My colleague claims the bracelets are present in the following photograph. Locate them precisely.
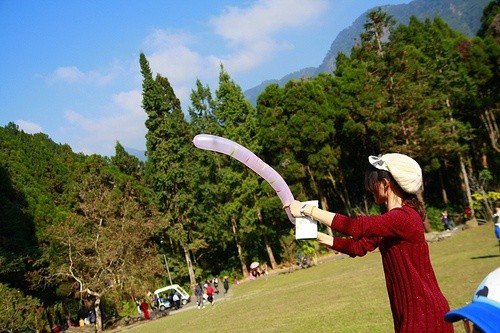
[310,204,316,221]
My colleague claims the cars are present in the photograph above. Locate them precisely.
[153,284,190,311]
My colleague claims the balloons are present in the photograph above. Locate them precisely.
[192,134,297,225]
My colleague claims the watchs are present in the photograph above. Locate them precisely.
[300,203,309,217]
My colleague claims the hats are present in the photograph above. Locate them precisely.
[445,267,500,333]
[368,153,424,193]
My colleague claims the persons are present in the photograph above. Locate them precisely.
[233,275,239,284]
[195,282,205,309]
[250,262,268,280]
[444,266,500,333]
[207,283,213,304]
[224,278,228,294]
[297,251,308,265]
[492,206,500,240]
[460,202,472,225]
[141,299,151,320]
[441,209,458,230]
[37,307,98,333]
[197,276,219,303]
[161,290,181,311]
[137,303,143,314]
[282,153,454,333]
